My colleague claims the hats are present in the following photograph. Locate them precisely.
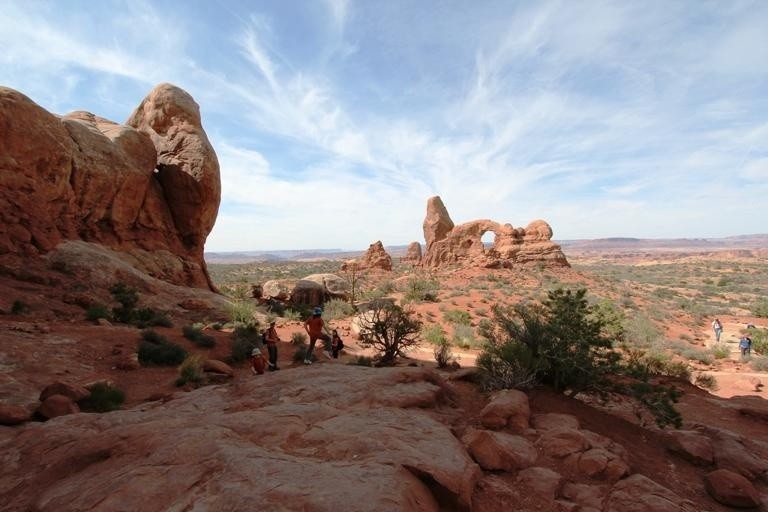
[251,348,262,356]
[313,307,322,315]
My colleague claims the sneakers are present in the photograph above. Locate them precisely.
[304,359,312,365]
[270,367,280,371]
[322,350,331,359]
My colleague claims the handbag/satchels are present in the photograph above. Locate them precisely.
[337,336,344,350]
[262,329,271,344]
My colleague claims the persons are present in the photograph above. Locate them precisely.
[739,334,752,356]
[264,323,281,370]
[711,318,724,342]
[303,308,331,365]
[330,330,340,360]
[248,349,274,376]
